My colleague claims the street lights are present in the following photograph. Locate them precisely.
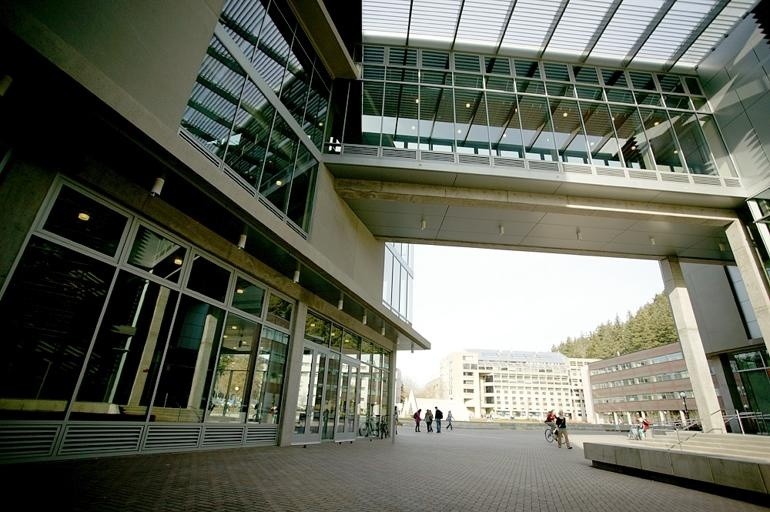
[680,392,690,425]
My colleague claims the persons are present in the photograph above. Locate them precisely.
[395,406,455,434]
[555,410,573,449]
[545,410,559,441]
[635,412,646,440]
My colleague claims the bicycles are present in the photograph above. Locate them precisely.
[545,424,566,444]
[359,414,389,437]
